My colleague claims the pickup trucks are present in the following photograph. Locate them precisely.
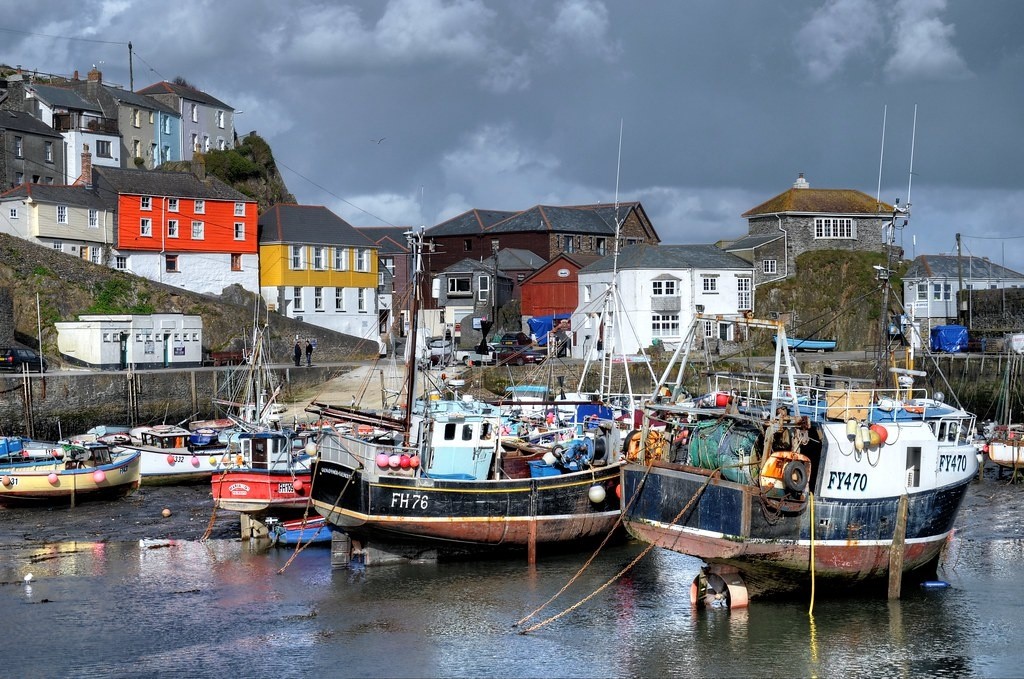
[453,342,503,367]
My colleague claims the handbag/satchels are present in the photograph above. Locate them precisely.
[292,355,296,361]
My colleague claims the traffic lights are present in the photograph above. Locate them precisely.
[446,330,451,341]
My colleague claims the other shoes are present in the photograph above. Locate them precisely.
[295,364,300,366]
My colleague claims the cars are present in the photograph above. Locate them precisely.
[500,332,533,348]
[0,347,49,375]
[489,345,547,367]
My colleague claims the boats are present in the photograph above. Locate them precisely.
[210,290,430,541]
[619,105,983,609]
[307,230,697,569]
[0,291,297,511]
[263,514,332,548]
[772,333,837,350]
[301,221,1024,482]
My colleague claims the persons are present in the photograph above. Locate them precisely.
[295,342,302,366]
[589,414,600,429]
[306,341,313,367]
